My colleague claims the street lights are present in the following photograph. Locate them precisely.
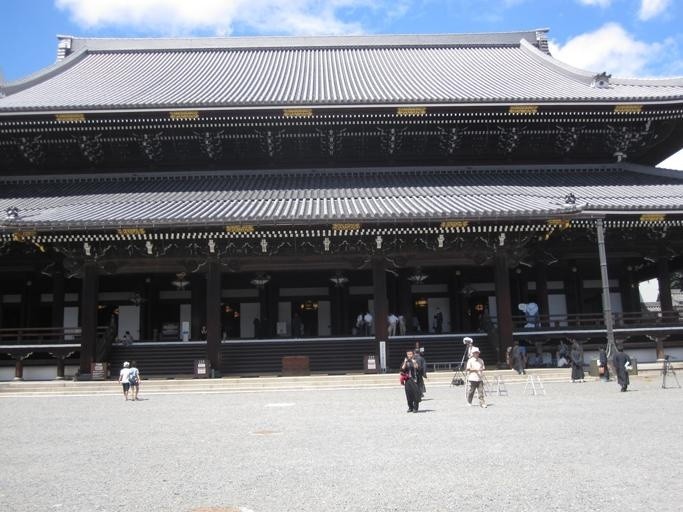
[598,367,605,374]
[128,376,138,385]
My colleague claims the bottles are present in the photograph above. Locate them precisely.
[123,361,130,367]
[472,347,481,354]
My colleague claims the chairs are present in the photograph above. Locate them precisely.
[466,401,473,407]
[412,408,418,413]
[480,404,487,408]
[406,408,413,412]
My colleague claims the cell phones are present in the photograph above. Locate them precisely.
[406,359,412,362]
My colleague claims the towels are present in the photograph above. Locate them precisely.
[363,350,379,374]
[193,358,211,379]
[90,360,108,381]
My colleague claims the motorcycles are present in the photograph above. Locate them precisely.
[451,344,468,387]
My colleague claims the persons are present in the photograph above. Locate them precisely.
[464,347,488,408]
[126,331,133,346]
[123,335,127,344]
[524,299,540,327]
[400,350,420,413]
[354,307,443,336]
[119,361,130,400]
[201,325,208,340]
[505,334,632,392]
[412,347,426,402]
[129,360,141,401]
